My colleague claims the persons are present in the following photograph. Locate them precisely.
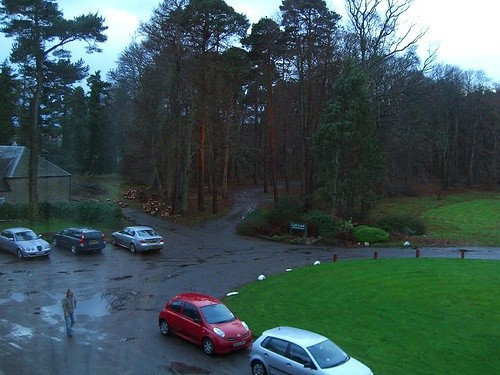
[62,288,77,337]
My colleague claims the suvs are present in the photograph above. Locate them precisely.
[52,227,106,256]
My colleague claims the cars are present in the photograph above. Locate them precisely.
[0,227,51,258]
[159,292,252,356]
[111,226,164,254]
[248,326,373,375]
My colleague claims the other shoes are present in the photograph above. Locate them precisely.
[67,333,72,338]
